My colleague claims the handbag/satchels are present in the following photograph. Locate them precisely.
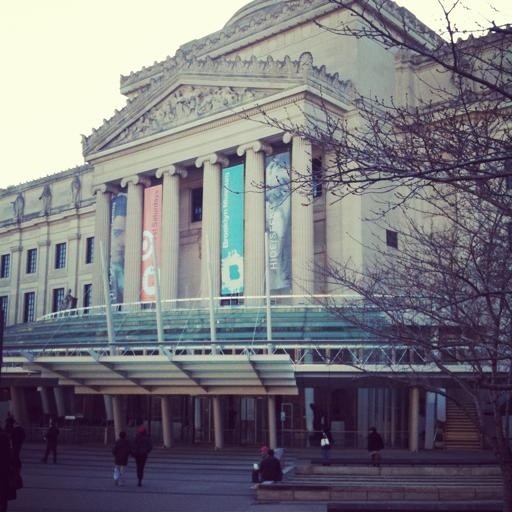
[116,440,139,457]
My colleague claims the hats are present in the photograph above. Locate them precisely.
[257,447,270,453]
[136,425,145,433]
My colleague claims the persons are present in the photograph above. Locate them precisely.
[0,430,23,511]
[132,424,152,486]
[367,427,385,466]
[112,431,129,487]
[4,411,13,439]
[11,423,25,450]
[320,424,332,466]
[309,401,325,430]
[41,418,60,464]
[260,449,283,482]
[248,445,268,491]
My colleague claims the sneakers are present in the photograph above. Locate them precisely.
[115,478,142,488]
[249,485,259,490]
